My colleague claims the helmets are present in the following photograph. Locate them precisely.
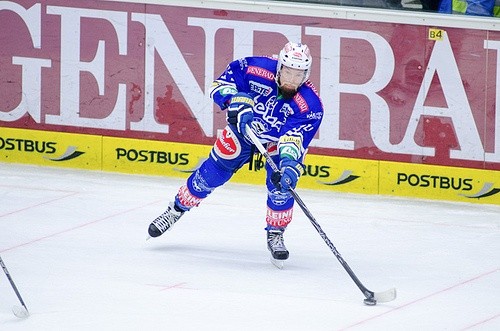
[278,42,312,85]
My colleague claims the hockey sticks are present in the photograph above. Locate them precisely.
[245,123,396,302]
[0,257,30,317]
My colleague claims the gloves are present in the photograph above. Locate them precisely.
[226,93,255,137]
[269,161,304,192]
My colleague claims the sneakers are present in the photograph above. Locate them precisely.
[263,226,290,269]
[146,204,185,237]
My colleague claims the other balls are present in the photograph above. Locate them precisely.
[364,299,376,305]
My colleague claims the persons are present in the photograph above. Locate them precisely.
[146,42,324,270]
